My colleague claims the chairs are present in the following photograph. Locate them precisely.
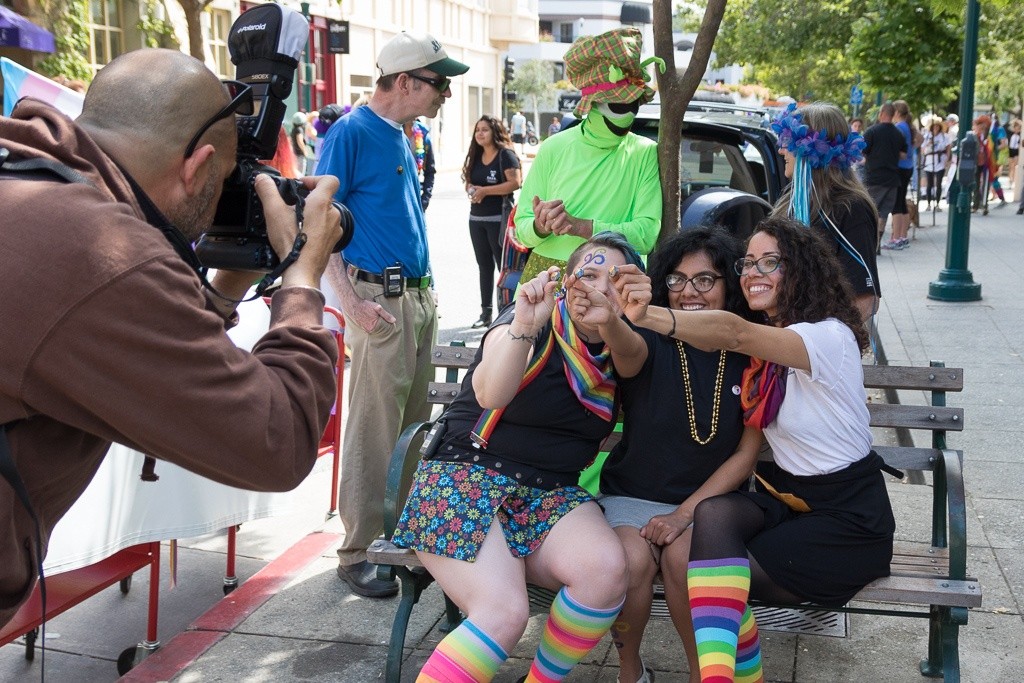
[731,160,770,204]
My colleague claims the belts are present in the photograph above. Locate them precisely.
[343,259,432,288]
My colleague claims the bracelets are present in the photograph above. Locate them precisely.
[666,307,677,338]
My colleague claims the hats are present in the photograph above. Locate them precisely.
[319,103,344,123]
[375,30,470,76]
[293,112,306,125]
[563,28,656,121]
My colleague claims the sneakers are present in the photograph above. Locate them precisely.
[882,239,903,250]
[900,238,910,247]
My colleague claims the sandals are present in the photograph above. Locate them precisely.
[617,656,654,683]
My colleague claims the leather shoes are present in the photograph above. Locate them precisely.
[337,559,399,598]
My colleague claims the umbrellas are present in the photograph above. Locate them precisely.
[0,4,55,54]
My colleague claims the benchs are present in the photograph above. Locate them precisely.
[366,340,983,683]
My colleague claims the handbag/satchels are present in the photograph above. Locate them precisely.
[498,197,514,247]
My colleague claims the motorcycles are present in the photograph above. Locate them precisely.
[526,129,538,146]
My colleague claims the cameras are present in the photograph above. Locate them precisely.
[196,3,356,270]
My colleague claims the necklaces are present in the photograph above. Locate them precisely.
[675,339,727,444]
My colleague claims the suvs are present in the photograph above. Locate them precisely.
[558,100,792,206]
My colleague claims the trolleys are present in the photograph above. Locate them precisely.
[0,541,160,682]
[118,297,346,600]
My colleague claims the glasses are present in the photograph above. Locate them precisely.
[185,79,255,159]
[734,255,783,275]
[664,274,724,292]
[394,72,452,91]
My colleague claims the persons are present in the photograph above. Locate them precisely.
[766,100,888,366]
[459,113,523,331]
[507,25,662,500]
[563,221,762,683]
[0,48,346,631]
[313,29,472,599]
[847,99,1024,251]
[546,116,561,138]
[386,232,647,682]
[511,110,535,144]
[604,216,905,683]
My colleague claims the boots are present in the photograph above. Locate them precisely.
[473,305,492,327]
